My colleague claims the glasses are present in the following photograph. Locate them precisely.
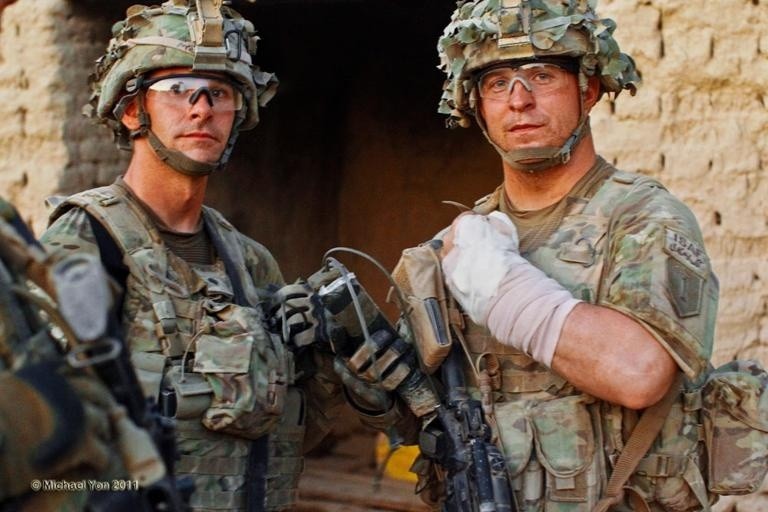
[139,73,246,110]
[475,62,579,102]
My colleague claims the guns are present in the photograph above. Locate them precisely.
[418,239,513,512]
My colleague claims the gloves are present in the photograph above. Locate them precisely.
[264,275,351,358]
[327,325,417,435]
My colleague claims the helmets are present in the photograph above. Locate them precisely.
[80,1,284,153]
[433,0,645,131]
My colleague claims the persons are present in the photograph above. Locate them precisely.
[328,0,721,508]
[0,196,195,512]
[34,0,327,512]
